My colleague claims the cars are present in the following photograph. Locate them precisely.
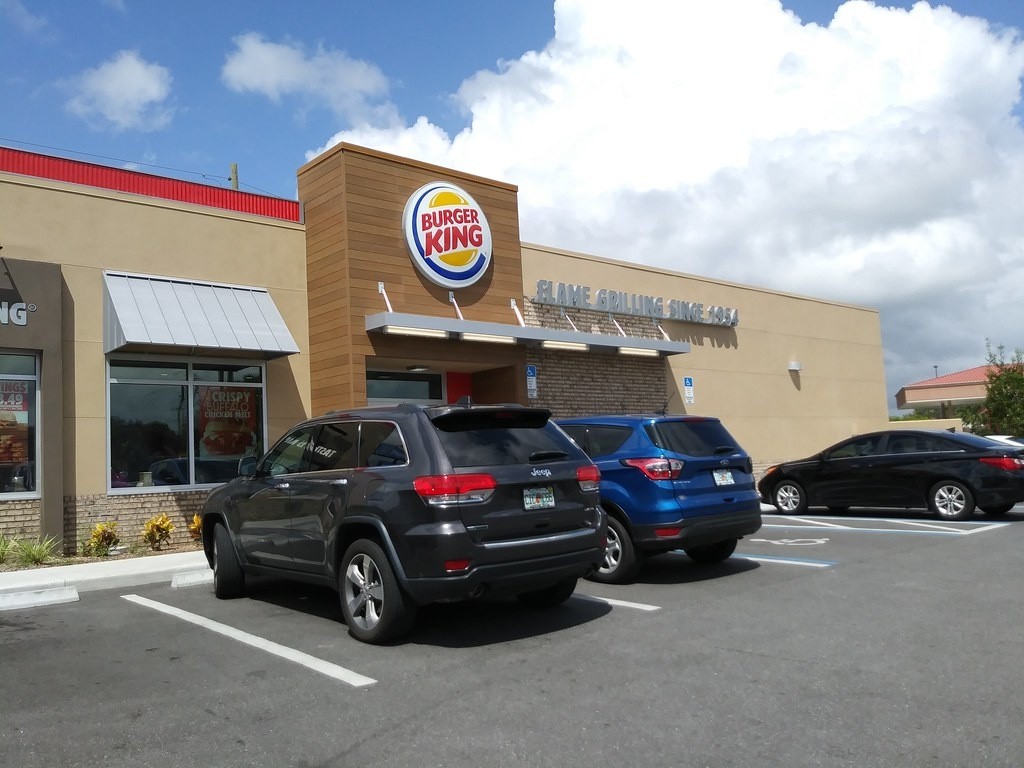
[757,425,1023,521]
[960,435,1024,454]
[549,414,761,583]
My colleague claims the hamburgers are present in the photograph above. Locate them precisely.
[0,411,26,462]
[202,417,253,455]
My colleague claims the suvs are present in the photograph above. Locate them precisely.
[199,397,605,645]
